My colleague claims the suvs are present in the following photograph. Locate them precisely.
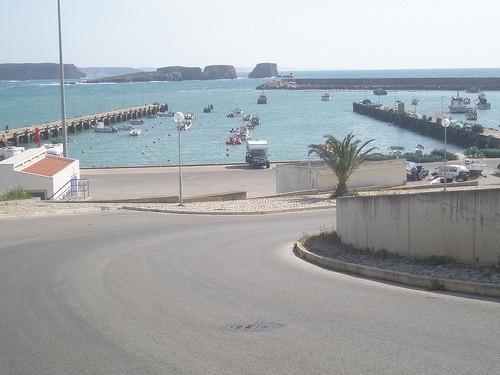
[431,165,470,181]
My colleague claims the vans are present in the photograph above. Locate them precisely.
[245,139,270,169]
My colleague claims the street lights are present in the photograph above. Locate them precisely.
[173,112,184,206]
[441,96,446,118]
[442,118,451,190]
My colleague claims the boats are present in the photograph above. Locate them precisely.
[415,143,425,154]
[320,93,330,101]
[373,86,387,95]
[411,97,418,105]
[390,145,405,150]
[448,89,470,113]
[226,108,259,144]
[146,111,173,119]
[257,91,267,104]
[464,106,477,120]
[95,119,144,136]
[179,112,199,130]
[465,86,491,109]
[202,104,215,113]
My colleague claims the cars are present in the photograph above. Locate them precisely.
[407,161,429,181]
